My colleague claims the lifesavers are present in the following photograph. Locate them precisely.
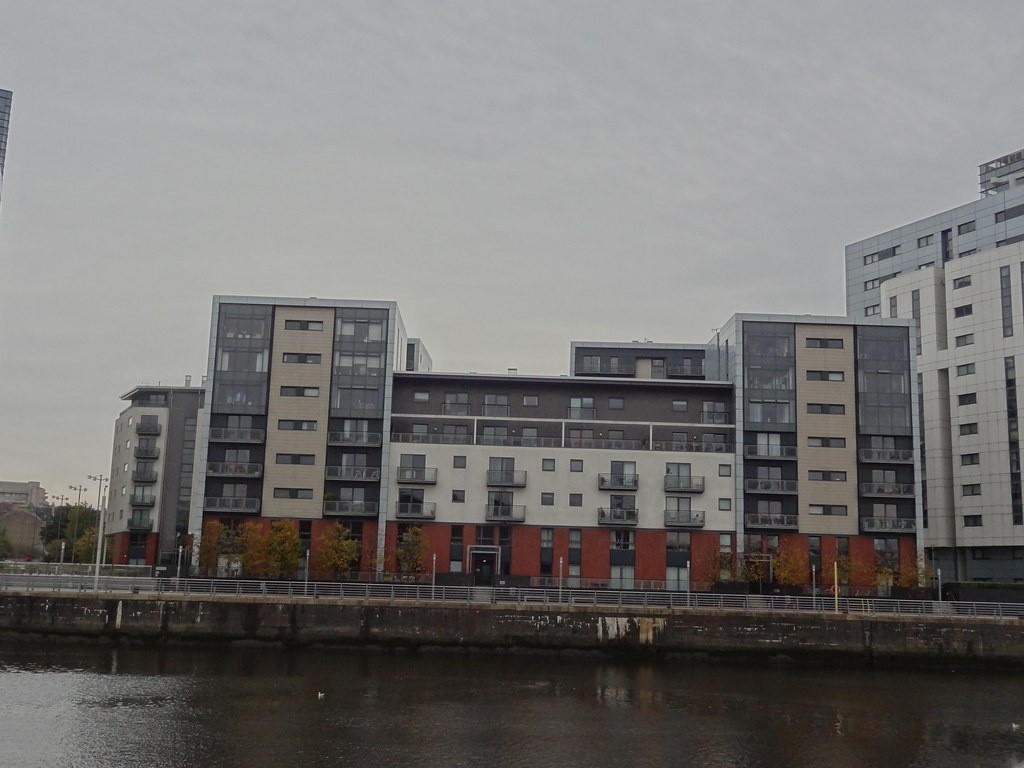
[830,584,841,594]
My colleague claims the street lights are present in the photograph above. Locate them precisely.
[69,484,87,563]
[304,549,310,596]
[176,545,183,592]
[52,494,68,562]
[687,561,690,607]
[938,569,941,601]
[558,556,563,604]
[30,508,44,558]
[58,542,65,590]
[812,564,816,611]
[88,475,109,563]
[432,553,436,600]
